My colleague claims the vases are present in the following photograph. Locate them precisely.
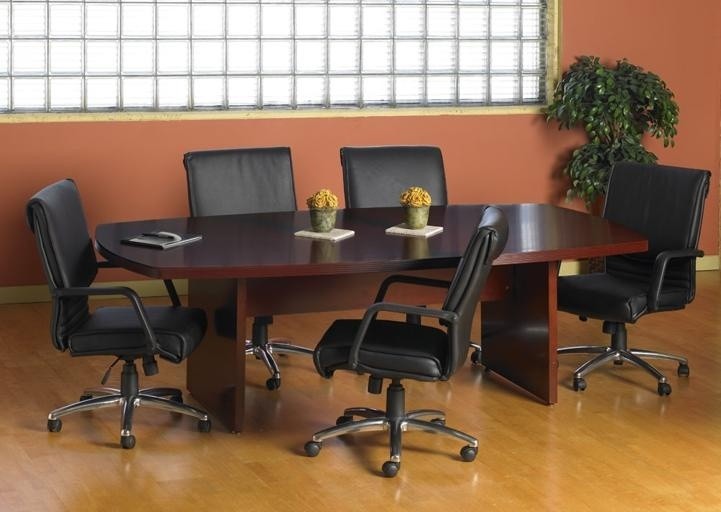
[404,205,429,229]
[310,208,337,232]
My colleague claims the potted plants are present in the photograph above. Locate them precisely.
[539,55,681,273]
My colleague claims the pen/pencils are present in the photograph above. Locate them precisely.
[142,231,175,239]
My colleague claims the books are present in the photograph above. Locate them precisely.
[122,230,202,251]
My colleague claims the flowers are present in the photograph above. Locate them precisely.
[307,188,337,209]
[400,186,432,208]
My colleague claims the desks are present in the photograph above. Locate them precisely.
[94,204,649,433]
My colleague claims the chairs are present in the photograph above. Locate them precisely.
[303,203,508,478]
[339,145,482,364]
[183,147,333,390]
[557,162,712,395]
[25,177,211,449]
